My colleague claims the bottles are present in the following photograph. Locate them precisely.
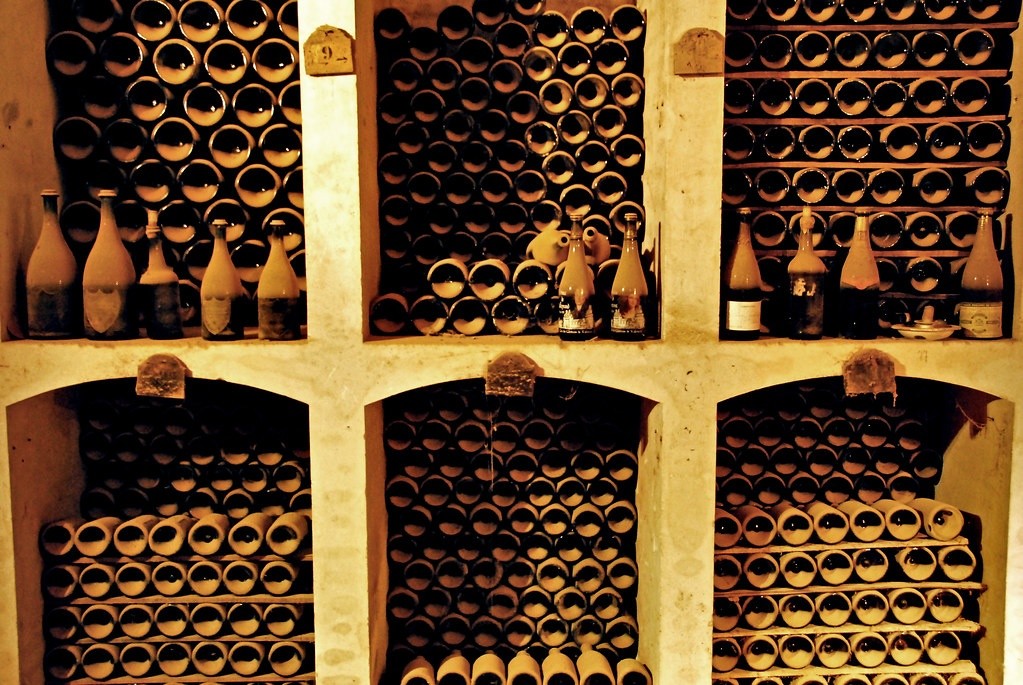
[17,2,1020,685]
